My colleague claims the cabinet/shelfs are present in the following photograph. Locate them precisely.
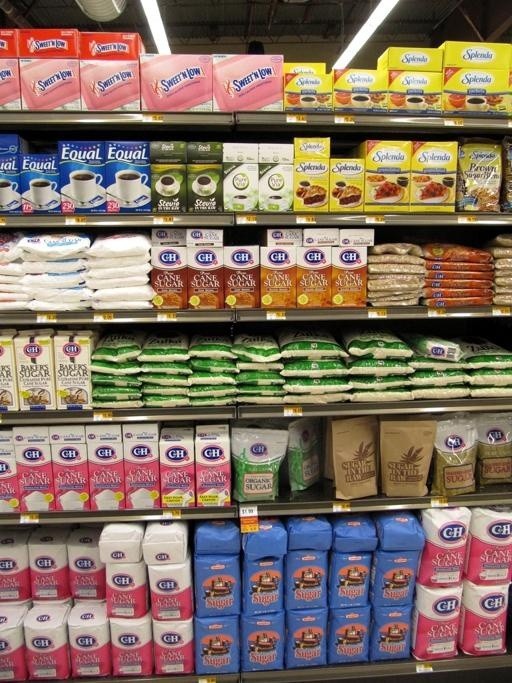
[0,109,510,682]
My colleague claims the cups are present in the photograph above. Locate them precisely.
[159,173,213,192]
[299,93,490,112]
[0,170,150,205]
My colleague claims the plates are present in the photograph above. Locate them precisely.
[154,180,217,198]
[1,185,152,212]
